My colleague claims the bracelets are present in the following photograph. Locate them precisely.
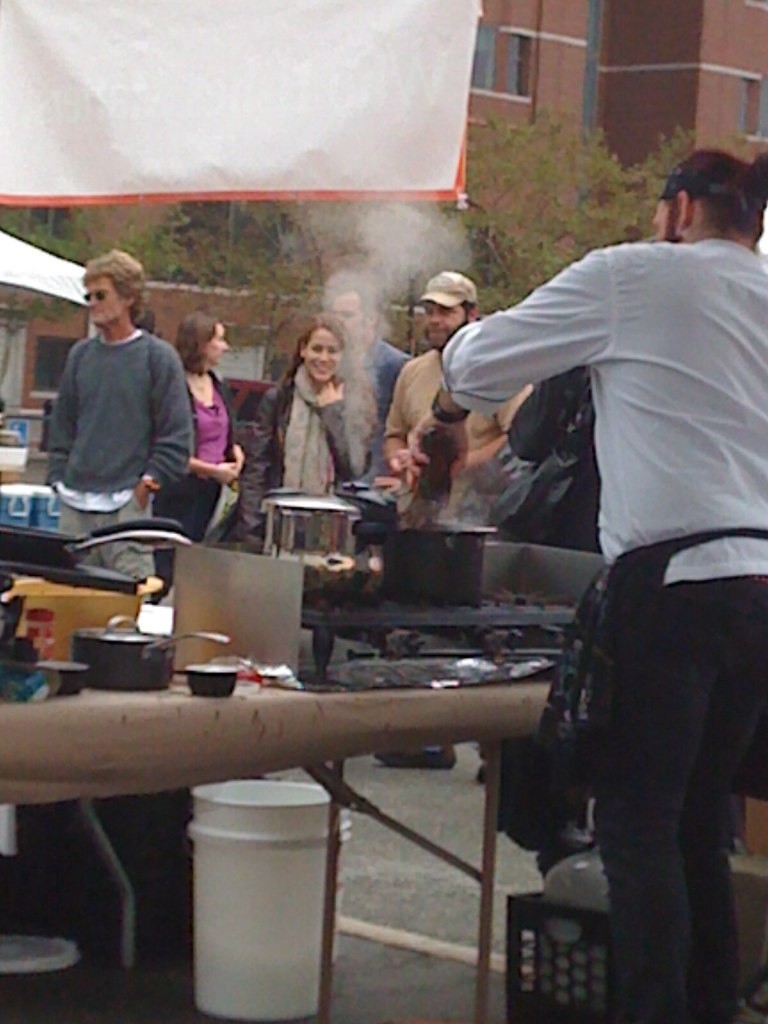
[431,392,470,423]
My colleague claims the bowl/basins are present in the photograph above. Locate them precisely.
[183,664,238,699]
[36,659,91,695]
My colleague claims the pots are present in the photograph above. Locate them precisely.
[0,517,193,568]
[261,493,373,592]
[352,520,500,606]
[72,615,230,691]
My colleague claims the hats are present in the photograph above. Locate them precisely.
[421,270,479,308]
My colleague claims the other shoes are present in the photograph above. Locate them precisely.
[375,745,456,769]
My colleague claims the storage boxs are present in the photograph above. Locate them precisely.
[0,484,61,534]
[504,888,610,1024]
[3,577,142,661]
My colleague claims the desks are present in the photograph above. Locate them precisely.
[0,668,550,1024]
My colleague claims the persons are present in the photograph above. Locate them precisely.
[145,311,245,604]
[47,250,193,601]
[321,272,413,479]
[407,150,768,1024]
[238,313,373,540]
[377,270,533,768]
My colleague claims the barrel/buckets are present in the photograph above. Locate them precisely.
[186,779,352,1021]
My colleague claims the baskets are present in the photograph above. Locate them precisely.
[505,893,611,1024]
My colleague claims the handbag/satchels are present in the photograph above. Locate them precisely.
[491,449,578,546]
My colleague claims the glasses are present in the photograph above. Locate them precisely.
[84,291,108,300]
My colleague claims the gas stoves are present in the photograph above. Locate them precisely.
[300,593,577,677]
